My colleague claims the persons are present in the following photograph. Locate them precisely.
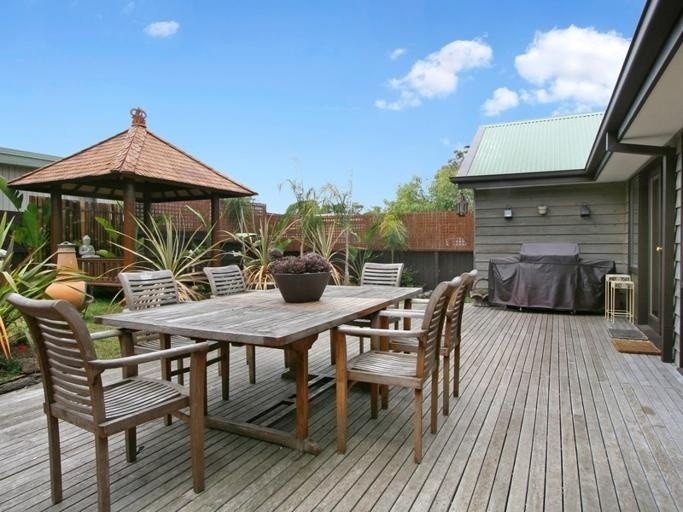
[78,235,101,259]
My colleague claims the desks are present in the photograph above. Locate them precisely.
[93,285,422,456]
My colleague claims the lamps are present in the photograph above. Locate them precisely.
[455,187,468,216]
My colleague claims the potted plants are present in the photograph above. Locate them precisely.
[266,252,333,303]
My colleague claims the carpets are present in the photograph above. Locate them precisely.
[606,327,662,355]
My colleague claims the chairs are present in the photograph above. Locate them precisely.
[119,268,230,427]
[8,291,210,512]
[341,261,405,359]
[379,268,478,416]
[204,265,275,386]
[334,276,462,463]
